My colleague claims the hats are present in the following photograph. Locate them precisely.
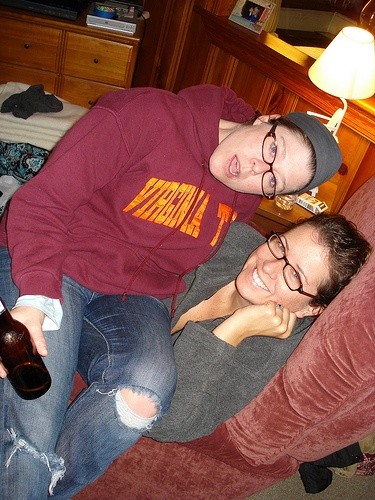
[282,112,342,195]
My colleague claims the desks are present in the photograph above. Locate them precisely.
[250,196,315,240]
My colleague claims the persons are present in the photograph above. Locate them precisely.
[246,6,259,23]
[140,211,371,443]
[0,84,343,500]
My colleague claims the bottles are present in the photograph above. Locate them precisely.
[0,299,52,401]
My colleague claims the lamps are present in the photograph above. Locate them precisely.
[307,26,375,196]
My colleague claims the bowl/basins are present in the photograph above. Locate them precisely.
[94,4,117,19]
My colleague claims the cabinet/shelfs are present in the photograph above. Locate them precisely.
[0,0,375,240]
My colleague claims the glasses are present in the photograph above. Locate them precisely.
[267,230,320,301]
[261,119,277,200]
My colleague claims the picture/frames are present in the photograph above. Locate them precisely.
[227,0,277,34]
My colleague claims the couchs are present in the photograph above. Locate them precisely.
[69,175,375,500]
